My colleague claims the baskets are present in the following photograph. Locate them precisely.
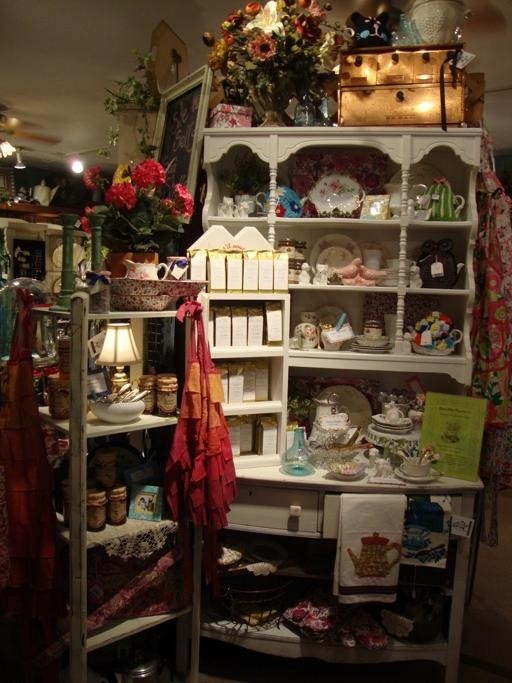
[225,581,289,632]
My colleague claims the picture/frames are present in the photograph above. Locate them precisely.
[127,480,164,523]
[151,64,210,227]
[83,365,113,402]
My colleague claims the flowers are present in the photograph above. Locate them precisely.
[201,0,360,111]
[76,155,194,251]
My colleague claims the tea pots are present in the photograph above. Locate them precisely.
[346,531,402,578]
[121,258,169,282]
[256,185,308,217]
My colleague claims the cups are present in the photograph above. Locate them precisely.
[386,258,411,268]
[385,407,403,420]
[364,320,383,336]
[399,456,432,477]
[300,311,317,322]
[365,249,381,268]
[387,183,411,219]
[218,194,255,217]
[321,331,343,351]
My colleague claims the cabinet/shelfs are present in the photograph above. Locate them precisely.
[24,293,207,683]
[195,126,484,365]
[186,223,295,471]
[336,43,468,126]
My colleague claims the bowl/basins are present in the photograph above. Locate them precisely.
[93,400,145,425]
[329,463,367,480]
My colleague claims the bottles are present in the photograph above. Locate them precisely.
[48,379,71,420]
[139,372,180,417]
[62,449,128,529]
[295,90,338,127]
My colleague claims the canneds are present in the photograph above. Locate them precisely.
[140,374,178,416]
[62,448,128,531]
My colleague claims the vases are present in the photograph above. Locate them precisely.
[102,254,160,279]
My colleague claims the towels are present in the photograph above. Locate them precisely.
[332,491,409,607]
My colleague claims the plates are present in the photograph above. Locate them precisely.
[308,172,363,217]
[378,273,410,287]
[309,233,363,276]
[372,414,413,434]
[309,384,372,435]
[346,335,392,353]
[395,462,442,483]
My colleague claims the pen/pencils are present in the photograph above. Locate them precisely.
[398,445,440,464]
[117,383,151,402]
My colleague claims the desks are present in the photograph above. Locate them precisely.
[189,448,487,683]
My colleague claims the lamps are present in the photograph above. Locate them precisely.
[12,145,26,170]
[93,320,143,394]
